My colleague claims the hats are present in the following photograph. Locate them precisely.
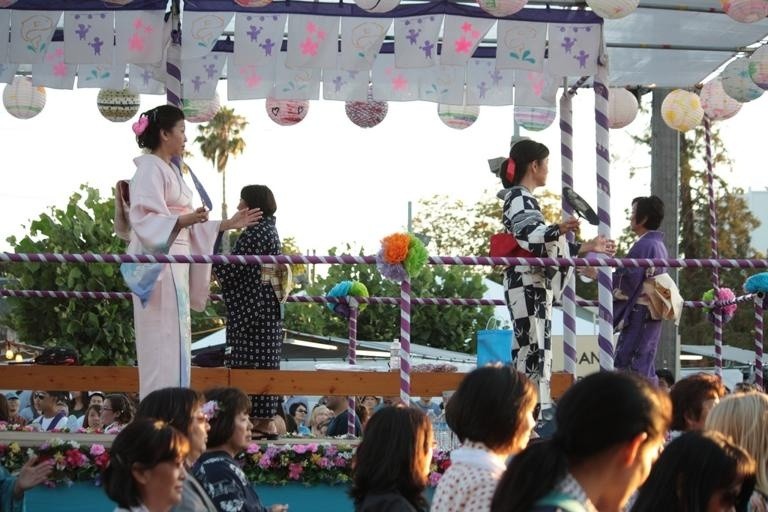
[6,391,18,399]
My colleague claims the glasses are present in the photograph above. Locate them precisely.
[297,409,307,414]
[35,392,43,399]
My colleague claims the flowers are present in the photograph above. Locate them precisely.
[426,442,454,489]
[1,441,110,488]
[236,442,351,488]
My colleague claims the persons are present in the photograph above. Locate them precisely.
[575,194,683,386]
[0,355,768,512]
[209,186,294,442]
[113,104,263,403]
[490,140,617,441]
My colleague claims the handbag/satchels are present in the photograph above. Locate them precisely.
[477,317,513,368]
